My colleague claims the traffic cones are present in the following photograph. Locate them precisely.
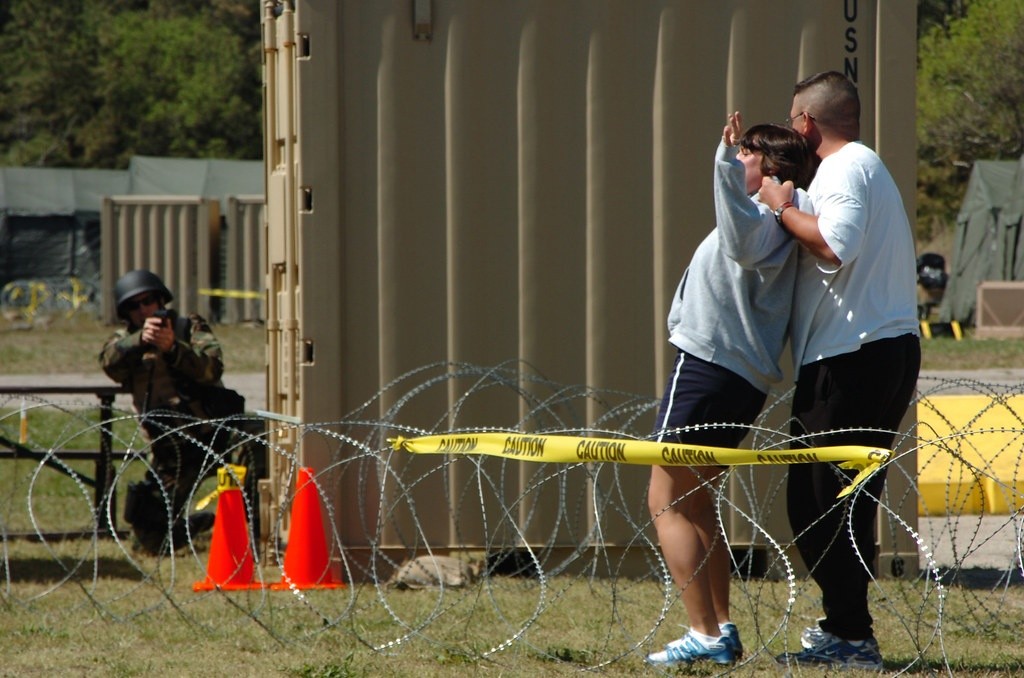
[193,463,270,591]
[270,466,344,591]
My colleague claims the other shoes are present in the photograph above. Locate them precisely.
[189,512,215,531]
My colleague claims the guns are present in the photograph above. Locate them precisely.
[150,308,179,330]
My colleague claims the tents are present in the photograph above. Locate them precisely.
[938,153,1024,324]
[0,153,267,322]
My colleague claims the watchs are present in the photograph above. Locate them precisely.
[775,201,793,224]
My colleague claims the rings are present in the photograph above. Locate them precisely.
[730,138,740,142]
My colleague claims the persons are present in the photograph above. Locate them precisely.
[644,108,812,666]
[758,70,921,671]
[98,270,267,553]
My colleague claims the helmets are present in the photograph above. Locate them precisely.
[114,270,173,320]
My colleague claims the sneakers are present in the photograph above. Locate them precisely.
[776,633,884,673]
[648,623,735,667]
[800,625,879,653]
[666,622,742,656]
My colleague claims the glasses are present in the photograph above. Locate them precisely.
[126,296,158,311]
[786,112,815,128]
